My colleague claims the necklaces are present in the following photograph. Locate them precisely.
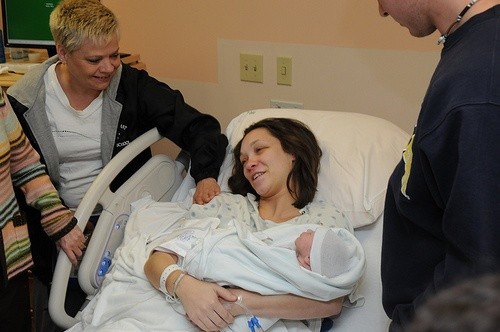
[438,0,479,44]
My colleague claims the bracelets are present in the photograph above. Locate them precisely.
[159,264,180,293]
[173,273,184,298]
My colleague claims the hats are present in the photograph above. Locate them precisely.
[311,226,366,290]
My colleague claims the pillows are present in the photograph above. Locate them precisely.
[177,109,412,228]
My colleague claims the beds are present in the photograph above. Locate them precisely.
[48,128,412,332]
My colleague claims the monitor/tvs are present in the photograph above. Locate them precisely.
[1,0,57,59]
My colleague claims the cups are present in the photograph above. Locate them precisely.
[29,53,42,62]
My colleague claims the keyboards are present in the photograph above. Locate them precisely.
[0,63,42,73]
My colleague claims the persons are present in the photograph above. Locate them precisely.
[4,0,228,332]
[0,85,85,332]
[143,117,353,332]
[176,221,366,302]
[377,0,500,332]
